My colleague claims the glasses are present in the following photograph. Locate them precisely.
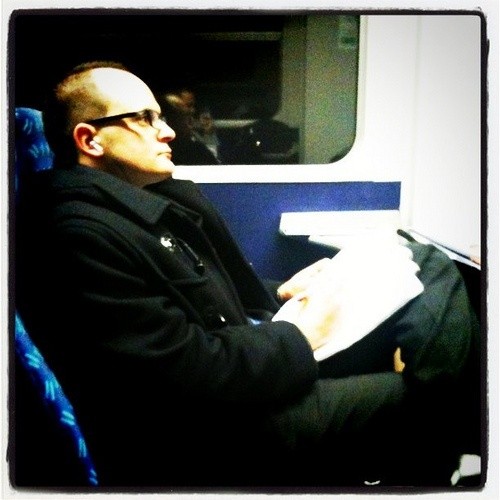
[176,107,194,122]
[87,109,172,131]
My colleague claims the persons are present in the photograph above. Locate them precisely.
[8,59,484,490]
[159,85,222,164]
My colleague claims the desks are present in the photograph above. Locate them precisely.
[308,230,481,275]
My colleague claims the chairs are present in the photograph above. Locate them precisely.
[8,104,99,490]
